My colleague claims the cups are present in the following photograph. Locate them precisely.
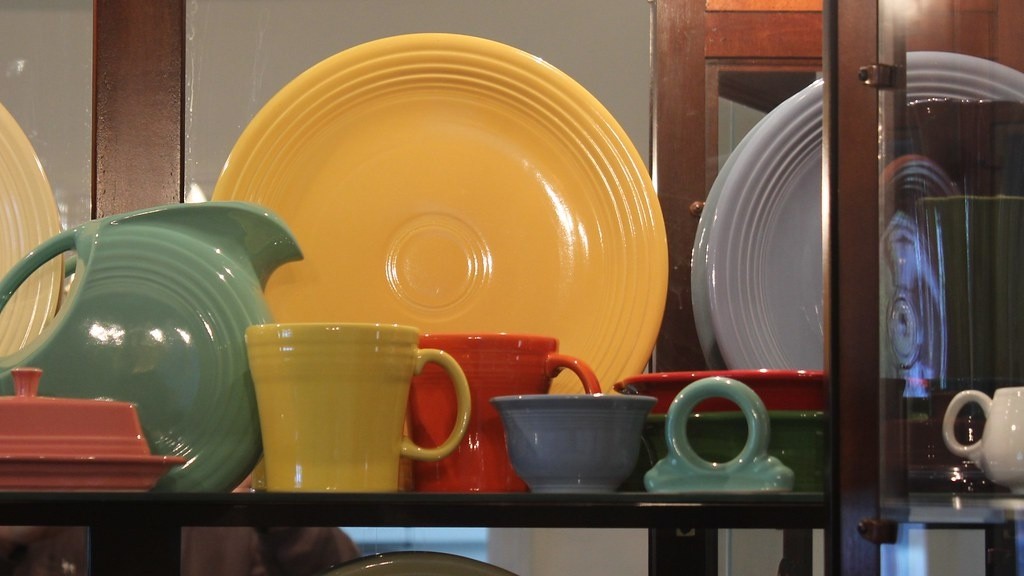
[410,333,602,493]
[491,398,658,494]
[244,323,470,493]
[942,386,1024,496]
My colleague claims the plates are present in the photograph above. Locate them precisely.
[0,101,64,360]
[690,51,1024,370]
[2,454,190,488]
[206,33,669,395]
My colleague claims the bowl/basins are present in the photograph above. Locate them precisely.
[614,372,831,493]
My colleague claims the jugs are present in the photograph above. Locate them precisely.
[1,200,305,490]
